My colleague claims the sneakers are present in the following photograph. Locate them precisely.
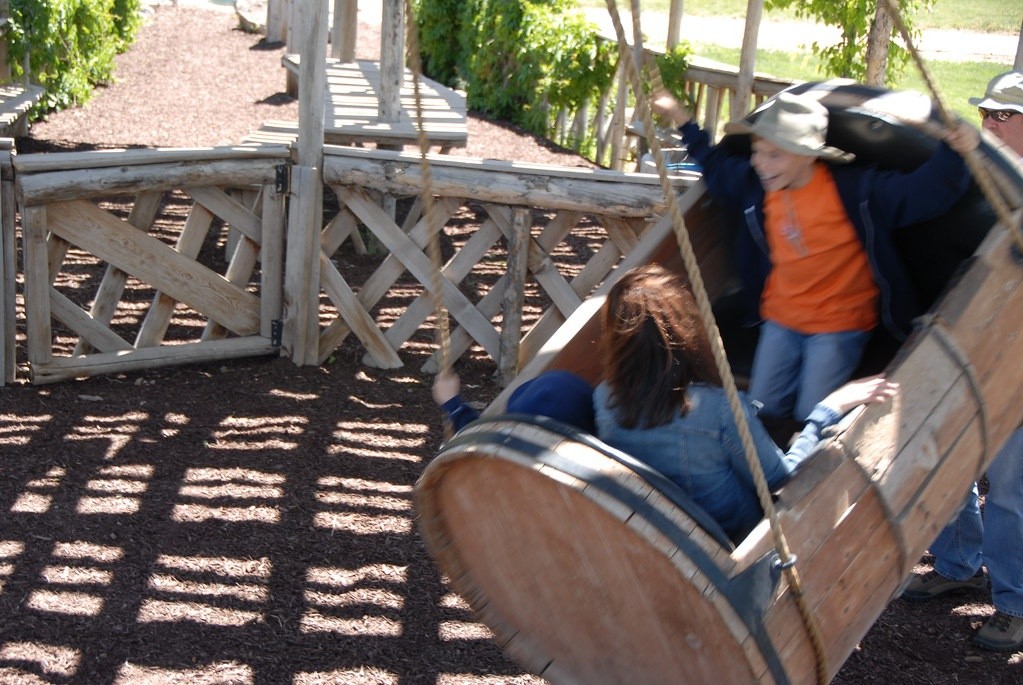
[901,567,987,602]
[970,610,1022,651]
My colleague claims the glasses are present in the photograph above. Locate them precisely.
[978,107,1020,122]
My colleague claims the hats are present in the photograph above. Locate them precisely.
[967,72,1023,111]
[723,91,854,164]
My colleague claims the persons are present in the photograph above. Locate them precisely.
[644,90,982,427]
[430,262,899,549]
[901,71,1023,653]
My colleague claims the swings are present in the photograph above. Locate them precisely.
[395,3,1022,682]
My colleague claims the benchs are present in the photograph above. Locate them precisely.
[225,119,299,262]
[281,54,468,150]
[0,83,48,140]
